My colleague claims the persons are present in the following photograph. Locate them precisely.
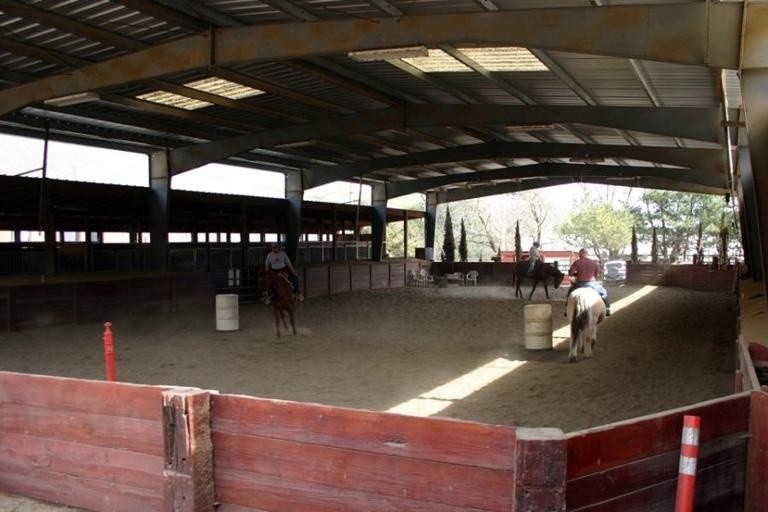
[264,244,304,305]
[526,242,541,277]
[564,248,610,316]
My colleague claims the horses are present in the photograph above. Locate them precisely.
[566,277,608,365]
[270,268,300,341]
[511,258,565,301]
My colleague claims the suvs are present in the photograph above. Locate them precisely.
[603,259,627,283]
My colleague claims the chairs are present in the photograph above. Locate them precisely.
[407,268,435,288]
[466,271,479,286]
[447,271,465,286]
